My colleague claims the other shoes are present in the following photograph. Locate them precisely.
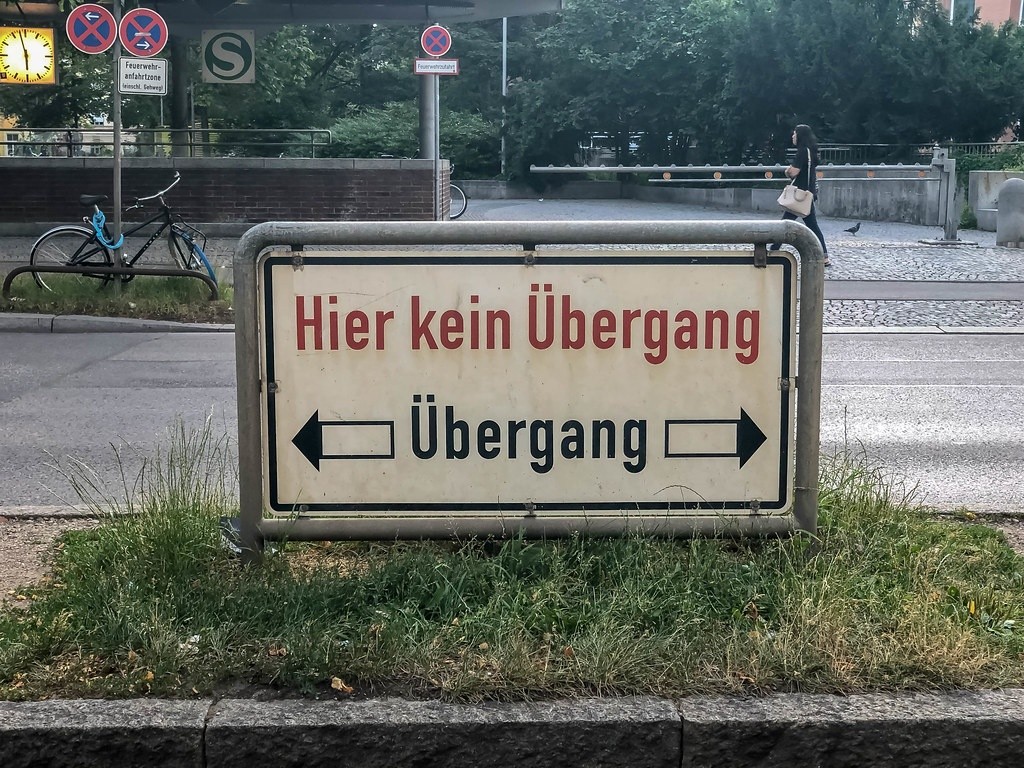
[824,258,831,266]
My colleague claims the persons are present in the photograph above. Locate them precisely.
[769,124,832,267]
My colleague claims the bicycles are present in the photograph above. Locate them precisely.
[29,169,219,302]
[381,149,467,219]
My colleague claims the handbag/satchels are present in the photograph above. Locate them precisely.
[777,185,813,218]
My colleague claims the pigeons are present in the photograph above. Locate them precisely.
[844,223,861,236]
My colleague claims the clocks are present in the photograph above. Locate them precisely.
[0,27,60,84]
